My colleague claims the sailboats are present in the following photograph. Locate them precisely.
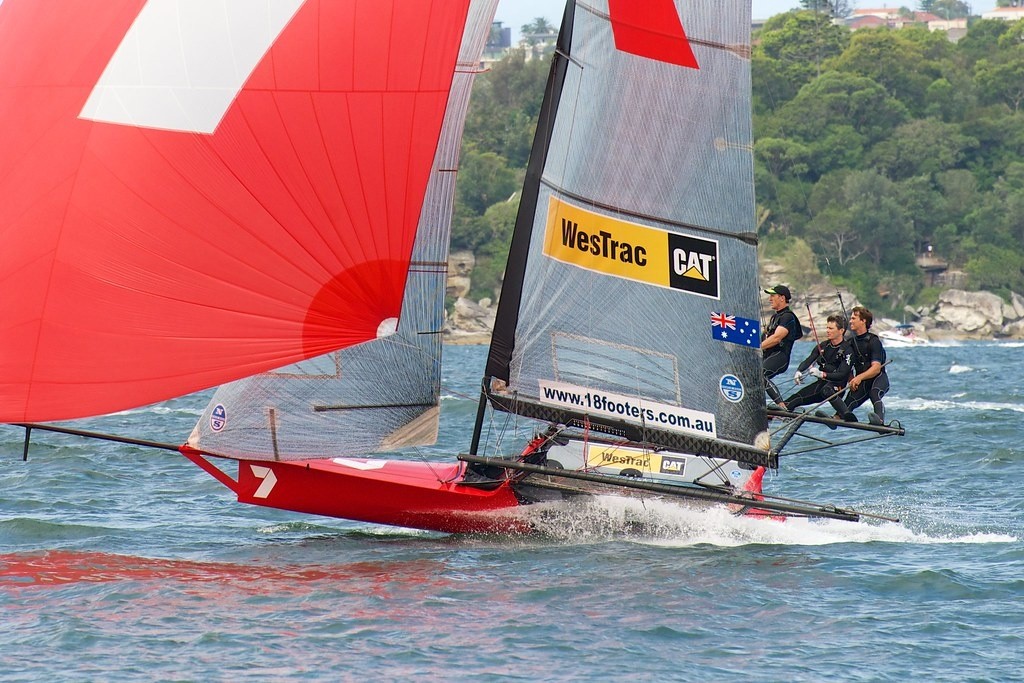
[0,1,907,544]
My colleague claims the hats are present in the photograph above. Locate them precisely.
[764,285,790,299]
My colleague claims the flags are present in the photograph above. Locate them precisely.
[711,309,760,349]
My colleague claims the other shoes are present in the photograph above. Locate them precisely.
[844,412,858,422]
[868,412,885,435]
[816,410,837,430]
[769,404,787,411]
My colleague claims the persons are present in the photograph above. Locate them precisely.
[775,316,860,423]
[816,307,891,435]
[761,285,804,410]
